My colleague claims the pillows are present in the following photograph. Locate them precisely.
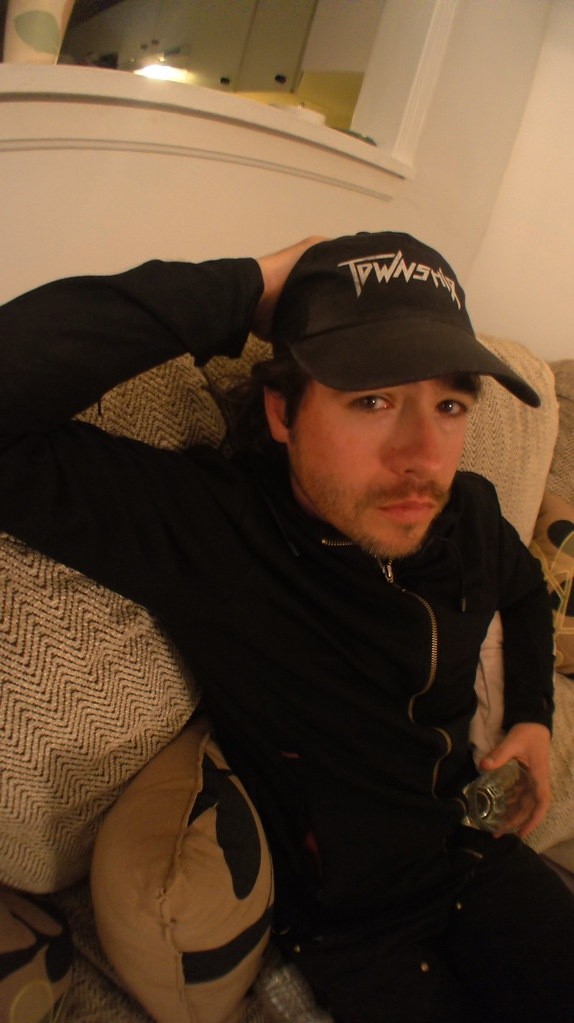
[527,488,574,682]
[90,713,276,1023]
[0,888,74,1023]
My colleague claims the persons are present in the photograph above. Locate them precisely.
[1,229,574,1023]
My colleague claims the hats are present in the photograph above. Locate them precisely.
[269,230,542,411]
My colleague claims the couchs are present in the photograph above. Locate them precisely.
[0,335,574,1023]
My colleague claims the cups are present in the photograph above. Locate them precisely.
[464,764,536,837]
